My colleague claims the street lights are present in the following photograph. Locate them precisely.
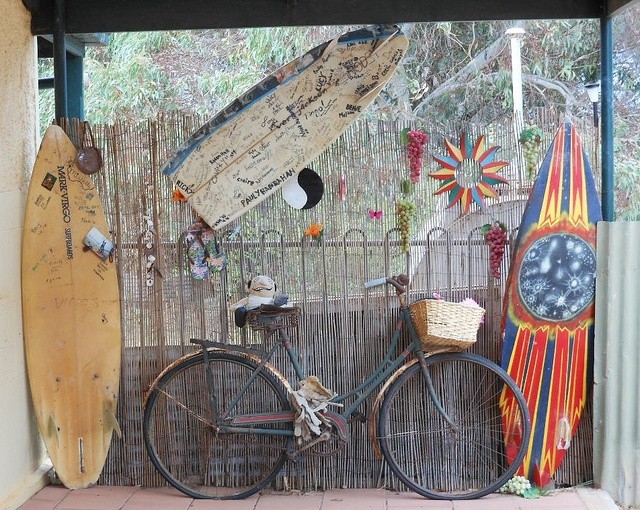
[585,83,599,189]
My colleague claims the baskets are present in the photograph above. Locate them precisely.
[408,299,485,353]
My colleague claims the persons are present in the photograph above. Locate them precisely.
[230,275,288,327]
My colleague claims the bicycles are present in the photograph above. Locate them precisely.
[143,275,529,499]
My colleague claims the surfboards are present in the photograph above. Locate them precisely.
[500,120,604,492]
[162,24,410,232]
[21,125,122,491]
